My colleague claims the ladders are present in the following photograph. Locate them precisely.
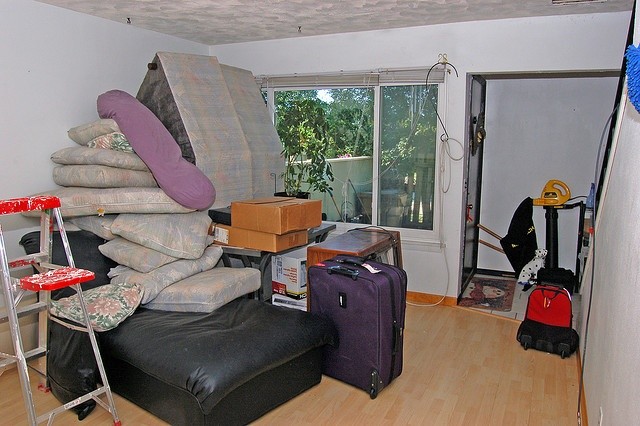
[0,195,124,426]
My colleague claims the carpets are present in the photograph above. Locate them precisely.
[459,276,516,312]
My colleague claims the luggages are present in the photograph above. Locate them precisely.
[306,253,408,400]
[516,279,580,359]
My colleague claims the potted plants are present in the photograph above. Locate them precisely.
[269,96,349,201]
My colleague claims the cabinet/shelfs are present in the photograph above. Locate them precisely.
[306,227,404,312]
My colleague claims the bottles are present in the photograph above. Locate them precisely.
[586,182,596,210]
[584,209,594,234]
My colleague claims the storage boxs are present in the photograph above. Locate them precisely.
[231,196,322,235]
[207,220,310,253]
[271,242,320,299]
[19,230,340,425]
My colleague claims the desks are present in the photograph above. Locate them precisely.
[219,223,337,302]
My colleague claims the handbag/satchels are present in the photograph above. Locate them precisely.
[535,265,576,295]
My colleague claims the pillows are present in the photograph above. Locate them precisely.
[87,131,133,152]
[107,245,224,305]
[97,236,214,273]
[65,118,120,146]
[50,145,150,172]
[110,213,214,260]
[67,214,117,240]
[95,88,217,212]
[52,165,158,189]
[49,282,145,333]
[141,267,262,314]
[21,185,197,217]
